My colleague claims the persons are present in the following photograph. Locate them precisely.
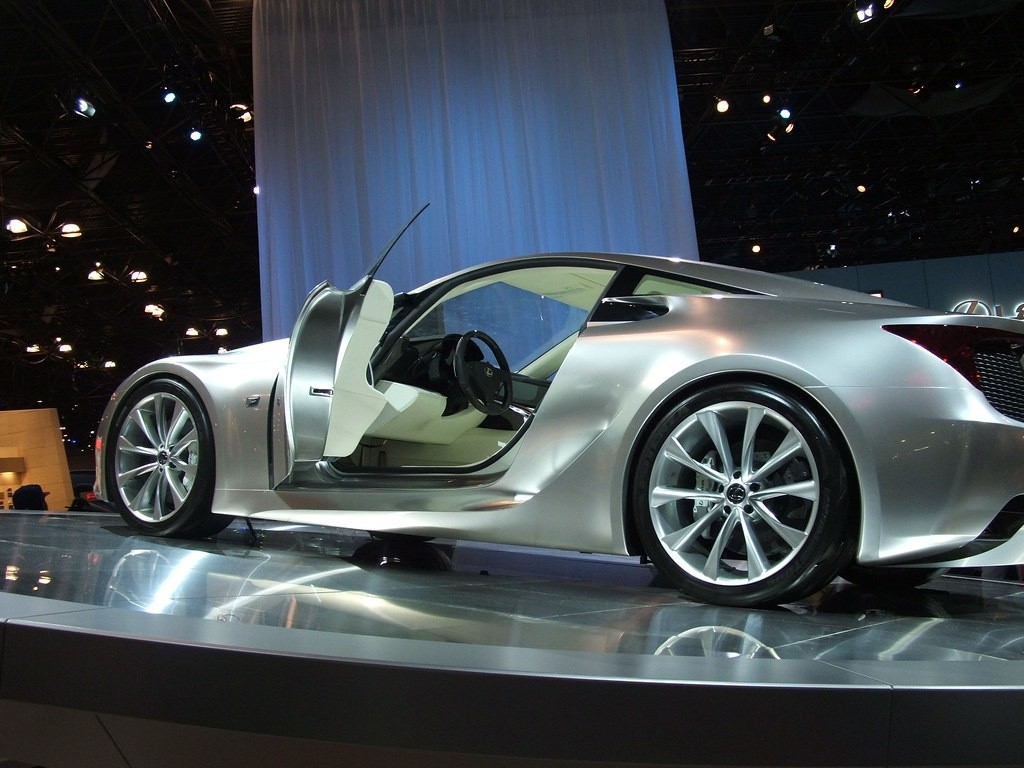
[12,484,49,510]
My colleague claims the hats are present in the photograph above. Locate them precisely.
[12,484,50,509]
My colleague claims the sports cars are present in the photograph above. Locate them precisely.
[92,206,1024,610]
